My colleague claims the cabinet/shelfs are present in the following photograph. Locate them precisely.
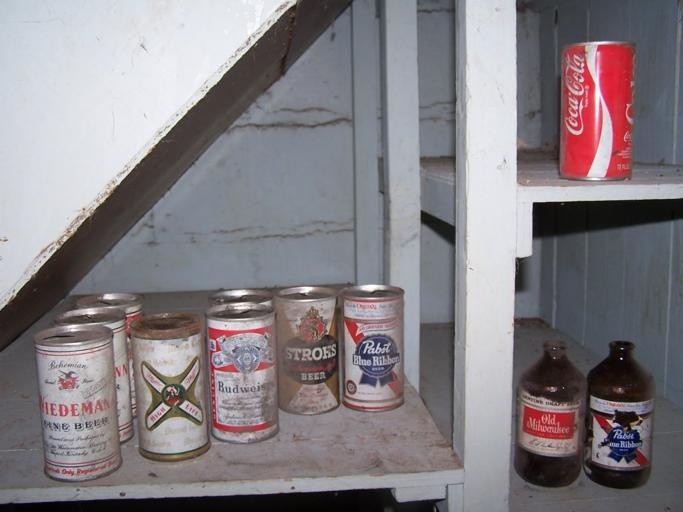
[380,2,681,508]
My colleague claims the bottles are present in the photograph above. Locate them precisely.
[516,339,657,490]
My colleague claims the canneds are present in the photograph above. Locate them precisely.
[272,285,341,416]
[338,283,405,413]
[557,40,634,181]
[129,312,211,462]
[50,292,146,444]
[203,288,280,444]
[33,324,124,482]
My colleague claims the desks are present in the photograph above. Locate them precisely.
[0,278,467,512]
